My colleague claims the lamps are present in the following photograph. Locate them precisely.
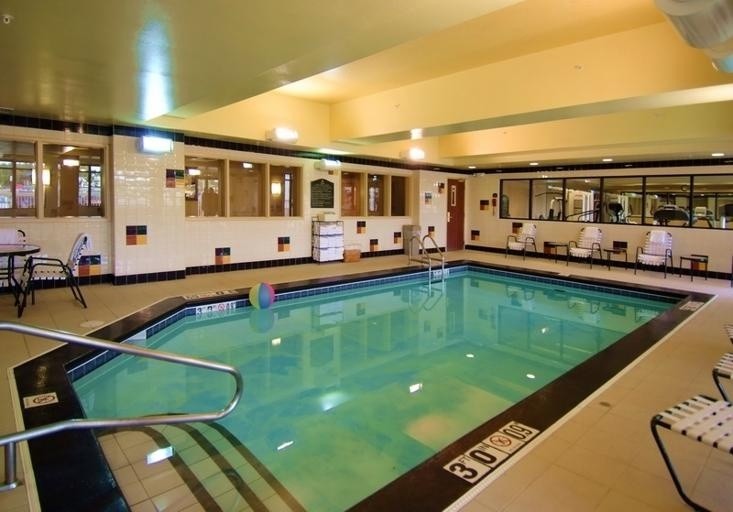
[135,134,174,155]
[263,126,299,146]
[709,50,732,77]
[314,159,341,171]
[663,1,732,50]
[398,146,426,162]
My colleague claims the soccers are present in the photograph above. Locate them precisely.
[249,283,275,309]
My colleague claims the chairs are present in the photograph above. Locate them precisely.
[503,223,537,259]
[567,227,604,269]
[650,324,733,512]
[632,228,676,280]
[0,228,90,321]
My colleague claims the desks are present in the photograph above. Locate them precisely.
[547,241,568,263]
[679,255,707,282]
[603,248,627,270]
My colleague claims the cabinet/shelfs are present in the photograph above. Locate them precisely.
[311,220,346,262]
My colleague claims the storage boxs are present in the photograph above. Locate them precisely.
[343,248,361,263]
